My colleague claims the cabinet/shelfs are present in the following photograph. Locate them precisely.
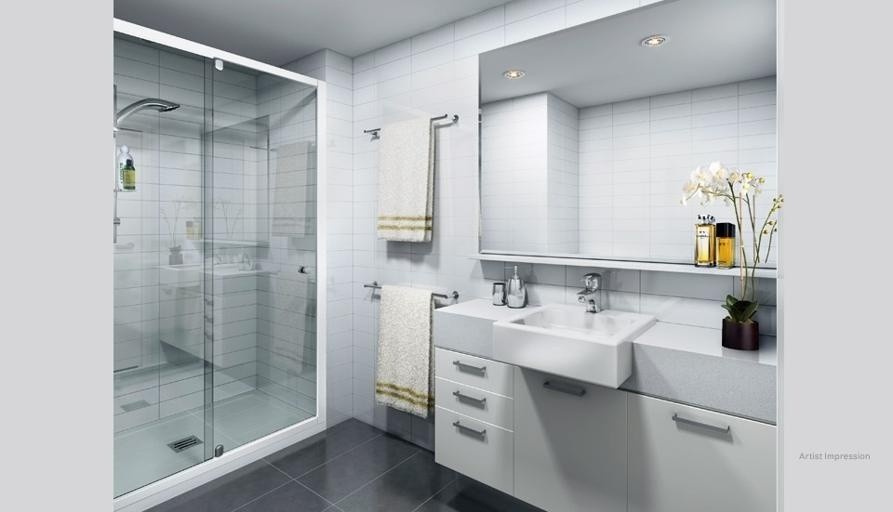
[433,347,514,497]
[513,366,628,511]
[628,391,777,512]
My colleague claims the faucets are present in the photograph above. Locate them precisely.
[578,272,602,313]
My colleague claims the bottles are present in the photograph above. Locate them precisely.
[122,158,134,190]
[694,224,715,269]
[185,221,192,240]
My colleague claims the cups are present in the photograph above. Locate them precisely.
[491,283,507,305]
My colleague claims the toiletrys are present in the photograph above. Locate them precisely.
[713,222,731,270]
[186,222,199,241]
[122,158,136,190]
[504,265,527,308]
[728,224,736,267]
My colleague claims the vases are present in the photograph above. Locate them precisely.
[722,319,759,351]
[168,253,183,265]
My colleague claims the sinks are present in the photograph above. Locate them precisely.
[156,263,201,285]
[492,305,656,390]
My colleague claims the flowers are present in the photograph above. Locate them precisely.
[678,159,784,321]
[739,170,777,264]
[160,194,186,253]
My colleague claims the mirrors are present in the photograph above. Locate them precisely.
[478,1,777,271]
[179,114,270,247]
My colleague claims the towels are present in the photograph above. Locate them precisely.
[376,116,435,244]
[375,285,436,419]
